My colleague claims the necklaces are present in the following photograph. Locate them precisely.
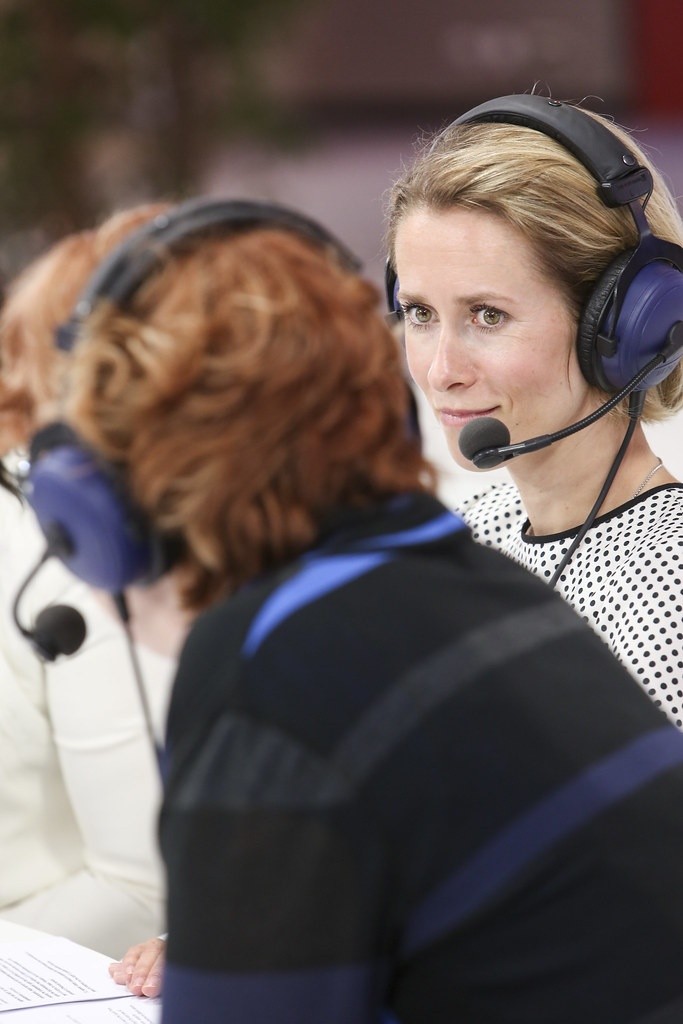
[634,456,663,497]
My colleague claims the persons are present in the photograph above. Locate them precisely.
[0,483,169,959]
[107,96,683,1000]
[0,196,683,1024]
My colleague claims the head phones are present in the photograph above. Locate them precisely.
[31,197,418,593]
[386,94,682,417]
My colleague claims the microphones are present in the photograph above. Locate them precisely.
[10,549,87,661]
[458,356,663,468]
